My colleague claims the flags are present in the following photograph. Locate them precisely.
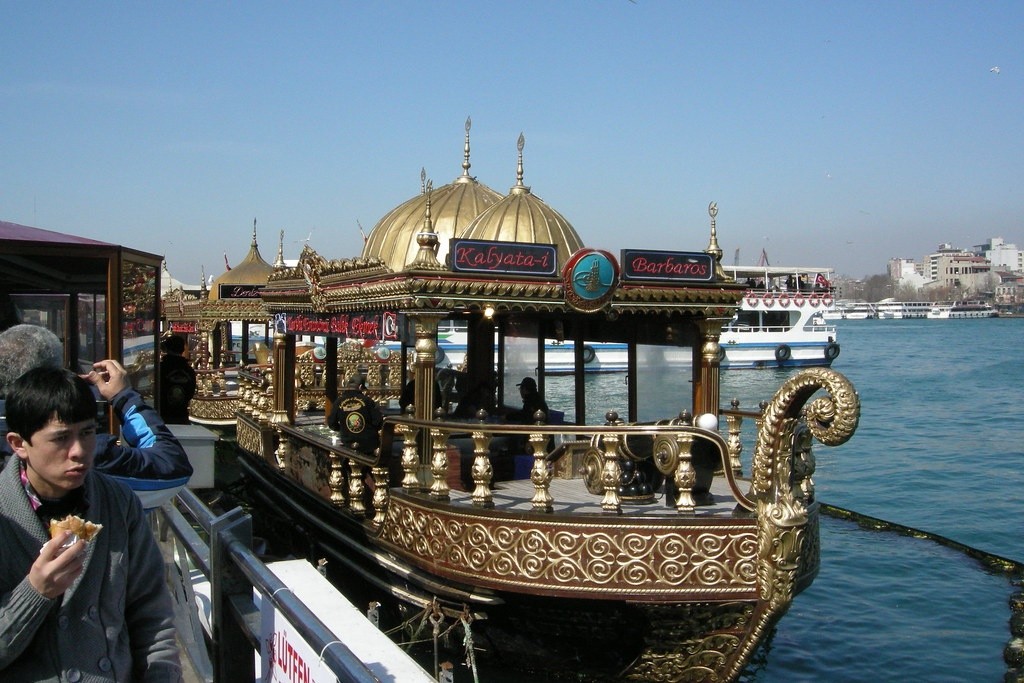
[816,273,828,287]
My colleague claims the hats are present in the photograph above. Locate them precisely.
[515,377,536,389]
[350,374,369,390]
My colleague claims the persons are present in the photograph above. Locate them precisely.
[746,274,805,290]
[329,375,383,457]
[0,324,194,515]
[450,381,504,418]
[399,377,441,418]
[0,366,185,683]
[495,378,548,482]
[160,335,196,424]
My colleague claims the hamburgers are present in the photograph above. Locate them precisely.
[48,514,102,543]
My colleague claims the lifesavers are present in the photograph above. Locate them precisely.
[778,293,790,307]
[762,293,774,306]
[822,293,834,306]
[793,293,805,307]
[775,344,791,361]
[808,293,820,306]
[747,293,759,307]
[582,344,595,363]
[824,343,840,359]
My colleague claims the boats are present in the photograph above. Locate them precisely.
[845,303,878,319]
[927,299,999,319]
[877,302,933,319]
[159,115,862,682]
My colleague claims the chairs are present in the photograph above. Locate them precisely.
[514,409,564,481]
